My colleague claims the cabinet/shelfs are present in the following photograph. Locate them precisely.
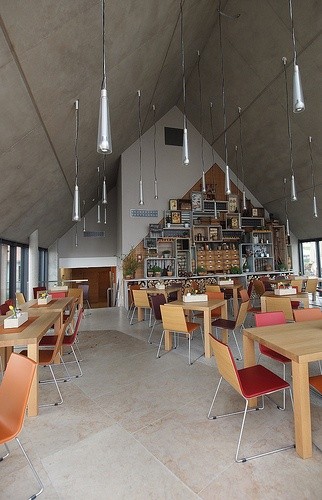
[144,190,291,279]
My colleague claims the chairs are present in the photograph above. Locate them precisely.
[0,283,93,500]
[127,285,322,462]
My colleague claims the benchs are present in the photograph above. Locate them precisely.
[145,288,181,302]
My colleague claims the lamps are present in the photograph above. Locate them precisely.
[70,0,318,247]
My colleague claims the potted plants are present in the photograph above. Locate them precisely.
[147,268,154,276]
[153,265,161,276]
[161,251,171,258]
[113,246,144,280]
[277,263,287,271]
[198,268,206,275]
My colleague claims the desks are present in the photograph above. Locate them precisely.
[242,319,322,460]
[219,284,243,319]
[260,290,310,313]
[271,280,291,285]
[165,298,228,358]
[0,312,61,417]
[5,296,74,329]
